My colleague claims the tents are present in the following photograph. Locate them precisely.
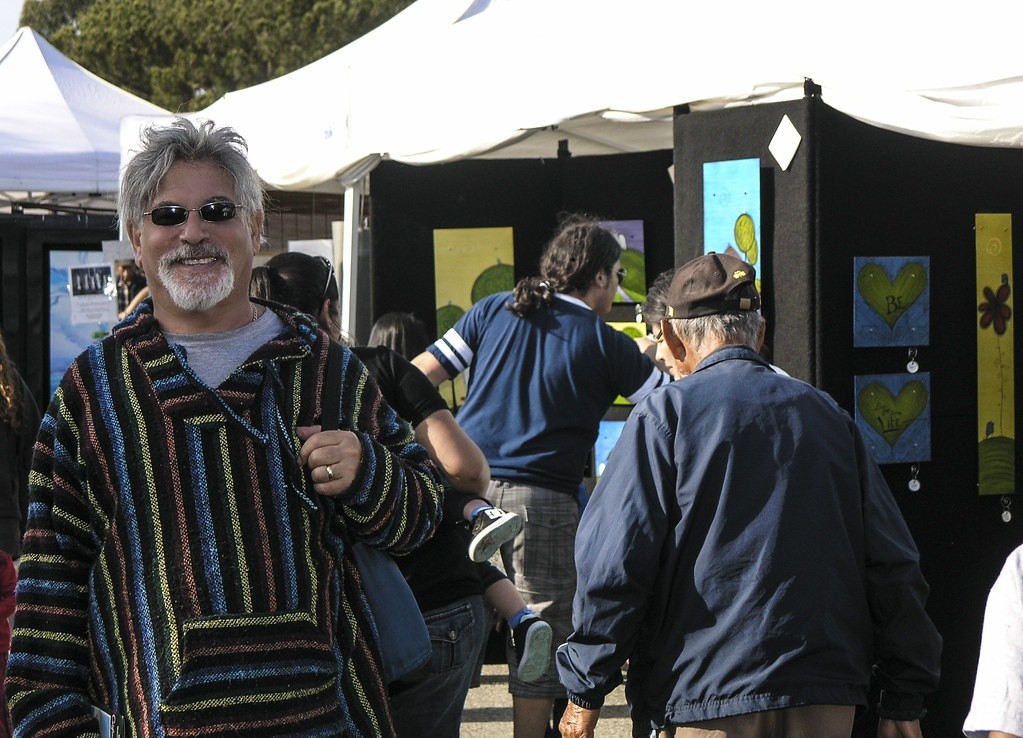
[0,26,175,193]
[118,0,1023,349]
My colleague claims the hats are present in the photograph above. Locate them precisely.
[665,252,761,318]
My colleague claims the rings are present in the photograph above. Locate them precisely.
[327,465,334,481]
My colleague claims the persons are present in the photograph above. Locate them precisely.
[410,223,674,738]
[248,252,553,738]
[962,543,1023,738]
[0,119,445,738]
[556,251,943,738]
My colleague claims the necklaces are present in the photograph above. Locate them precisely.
[250,303,258,322]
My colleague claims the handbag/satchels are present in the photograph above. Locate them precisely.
[345,539,432,680]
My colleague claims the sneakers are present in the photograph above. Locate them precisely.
[467,507,521,563]
[508,617,552,683]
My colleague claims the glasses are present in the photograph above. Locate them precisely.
[143,201,244,227]
[316,256,335,299]
[602,266,627,284]
